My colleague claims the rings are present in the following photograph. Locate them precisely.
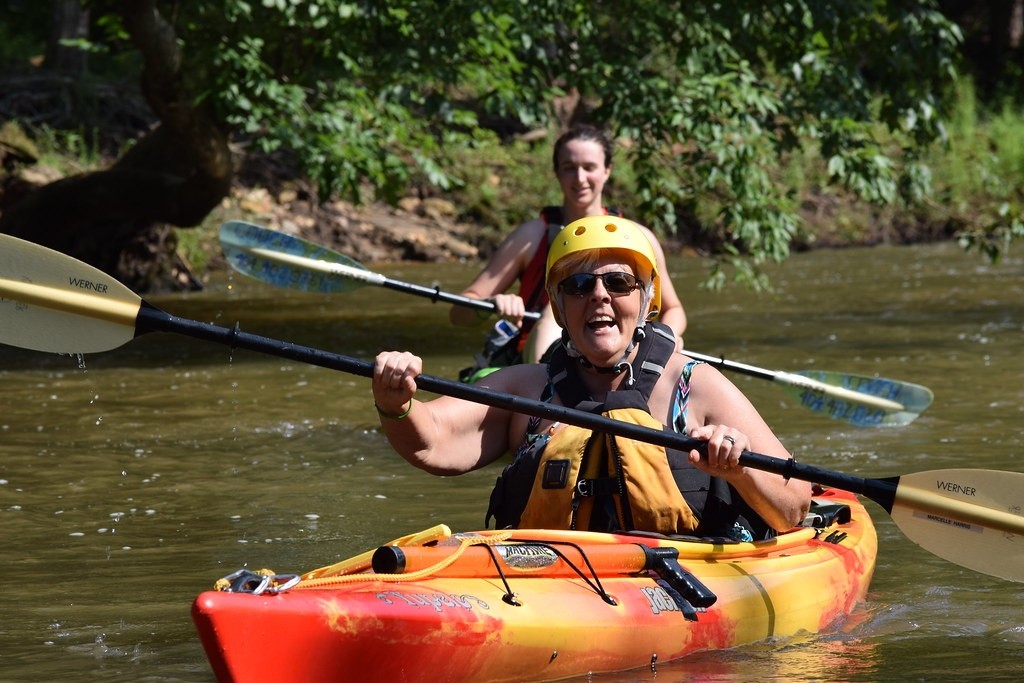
[723,434,736,446]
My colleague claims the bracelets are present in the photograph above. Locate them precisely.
[373,398,415,421]
[475,299,493,321]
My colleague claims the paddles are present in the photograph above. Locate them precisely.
[217,216,937,428]
[0,230,1023,589]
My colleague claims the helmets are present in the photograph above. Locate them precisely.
[545,215,662,331]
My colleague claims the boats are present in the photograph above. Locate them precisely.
[191,485,879,683]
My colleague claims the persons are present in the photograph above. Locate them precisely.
[373,124,812,543]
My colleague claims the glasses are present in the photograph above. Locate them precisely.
[557,271,644,296]
